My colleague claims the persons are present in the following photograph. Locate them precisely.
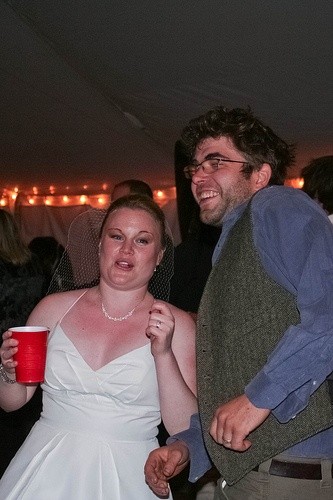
[143,105,332,500]
[0,209,51,481]
[0,193,198,500]
[110,180,152,198]
[298,156,333,225]
[27,234,74,294]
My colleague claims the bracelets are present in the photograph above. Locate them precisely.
[0,363,16,384]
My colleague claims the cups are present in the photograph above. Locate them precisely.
[9,326,50,385]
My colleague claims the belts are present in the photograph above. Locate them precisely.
[253,459,333,480]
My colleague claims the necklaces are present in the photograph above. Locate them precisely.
[99,296,141,322]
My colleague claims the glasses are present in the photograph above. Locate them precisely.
[182,159,252,180]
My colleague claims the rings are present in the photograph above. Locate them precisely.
[155,321,162,329]
[220,437,231,444]
